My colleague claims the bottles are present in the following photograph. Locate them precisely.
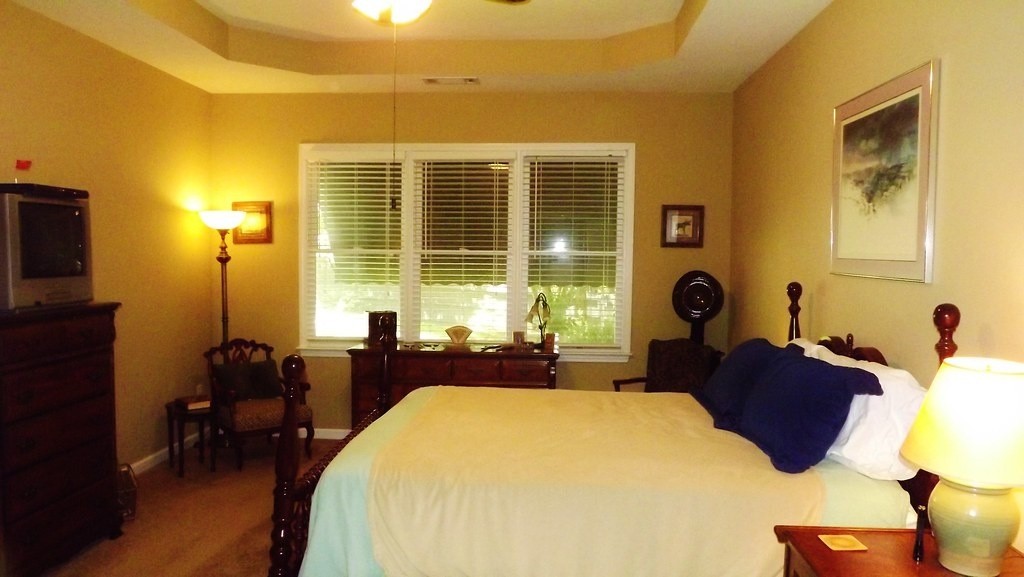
[11,159,32,183]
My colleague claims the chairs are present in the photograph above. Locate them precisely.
[202,338,315,469]
[612,269,725,393]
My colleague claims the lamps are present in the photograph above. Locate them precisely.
[525,292,551,353]
[201,210,243,342]
[350,0,433,213]
[899,357,1024,577]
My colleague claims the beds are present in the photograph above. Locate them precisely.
[270,282,962,577]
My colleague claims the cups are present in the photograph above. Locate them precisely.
[397,341,403,350]
[363,337,368,349]
[523,342,534,353]
[513,331,523,344]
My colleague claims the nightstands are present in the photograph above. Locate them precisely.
[774,524,1024,577]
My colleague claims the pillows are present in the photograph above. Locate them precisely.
[646,337,925,481]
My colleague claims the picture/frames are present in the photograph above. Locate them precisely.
[660,205,704,249]
[230,201,272,245]
[827,57,941,285]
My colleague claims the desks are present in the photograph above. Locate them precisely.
[165,401,217,477]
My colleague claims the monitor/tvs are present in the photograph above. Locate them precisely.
[0,193,94,314]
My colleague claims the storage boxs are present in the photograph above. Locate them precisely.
[117,464,140,522]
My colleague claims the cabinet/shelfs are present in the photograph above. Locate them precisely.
[347,342,560,430]
[0,301,123,577]
